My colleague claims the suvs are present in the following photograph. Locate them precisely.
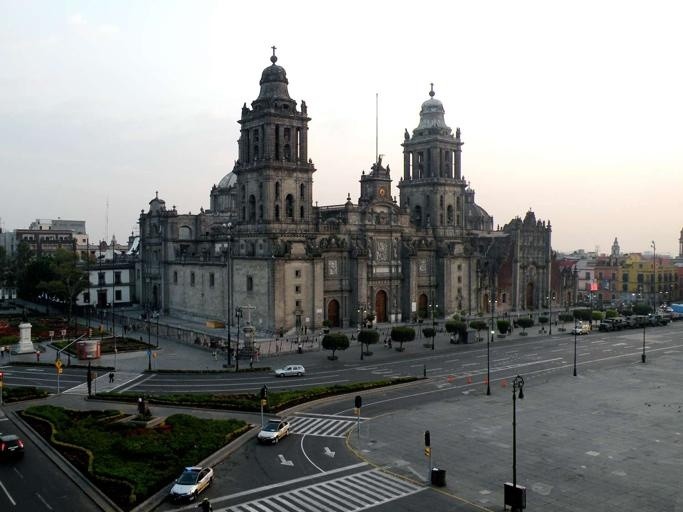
[0,433,26,459]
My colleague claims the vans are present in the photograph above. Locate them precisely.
[571,325,591,335]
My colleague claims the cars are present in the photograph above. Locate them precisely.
[275,364,305,378]
[169,465,215,501]
[258,418,293,446]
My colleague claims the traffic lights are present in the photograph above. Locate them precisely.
[88,328,92,338]
[354,396,360,407]
[261,388,267,399]
[423,430,430,447]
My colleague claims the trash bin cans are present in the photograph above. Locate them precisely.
[431,469,447,488]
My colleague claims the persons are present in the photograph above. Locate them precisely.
[124,322,136,335]
[0,346,5,356]
[109,372,114,383]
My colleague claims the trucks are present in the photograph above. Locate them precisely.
[599,315,670,332]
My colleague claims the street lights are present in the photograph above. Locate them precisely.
[649,239,659,316]
[428,299,439,350]
[587,291,596,330]
[545,290,557,335]
[510,369,530,511]
[221,219,238,367]
[657,288,672,305]
[233,303,243,373]
[153,313,162,350]
[484,319,500,395]
[630,287,641,314]
[488,293,497,342]
[356,301,368,361]
[571,308,584,375]
[640,309,650,364]
